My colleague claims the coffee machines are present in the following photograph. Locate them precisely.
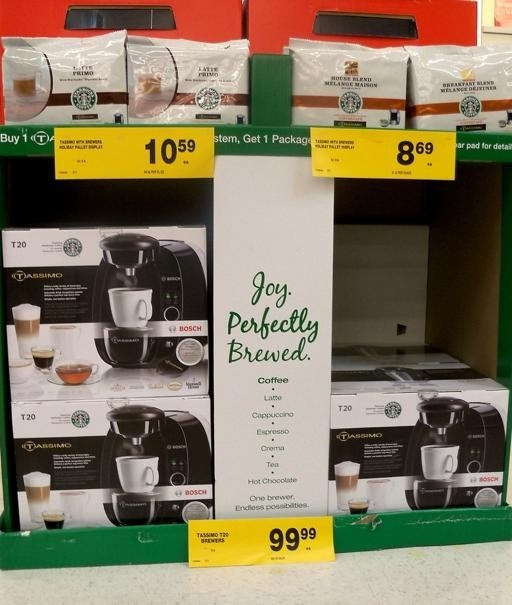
[92,233,206,370]
[403,397,505,510]
[99,404,211,527]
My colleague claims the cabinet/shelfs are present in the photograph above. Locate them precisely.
[1,52,511,570]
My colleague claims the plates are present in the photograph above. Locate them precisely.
[48,375,102,386]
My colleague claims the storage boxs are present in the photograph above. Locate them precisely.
[1,226,209,405]
[9,394,213,532]
[326,344,510,515]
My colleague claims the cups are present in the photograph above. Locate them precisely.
[42,510,65,529]
[23,474,52,524]
[108,287,154,330]
[420,444,460,481]
[115,455,160,494]
[31,344,56,369]
[348,497,369,514]
[55,364,99,385]
[365,479,394,515]
[59,489,89,529]
[334,463,361,512]
[12,306,42,360]
